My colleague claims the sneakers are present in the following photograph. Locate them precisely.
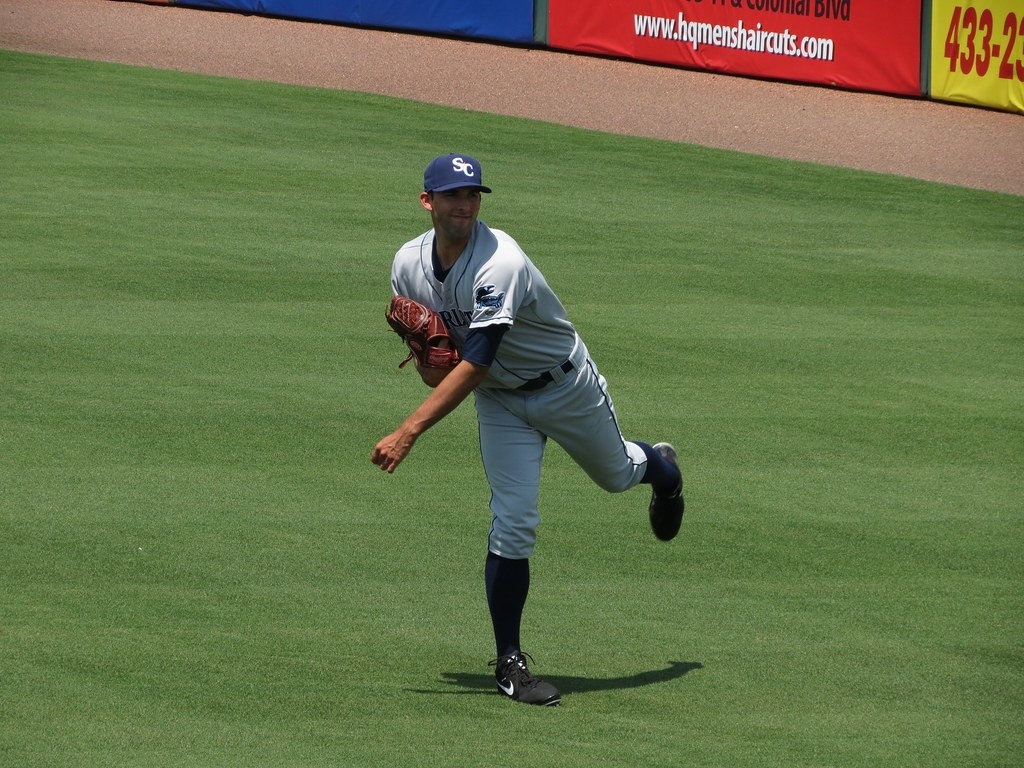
[488,650,562,707]
[649,442,684,541]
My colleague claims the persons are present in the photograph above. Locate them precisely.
[370,151,684,706]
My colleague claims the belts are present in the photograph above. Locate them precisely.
[519,360,574,391]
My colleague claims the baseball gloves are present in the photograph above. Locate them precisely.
[385,295,460,367]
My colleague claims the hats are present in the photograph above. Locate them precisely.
[424,153,492,193]
